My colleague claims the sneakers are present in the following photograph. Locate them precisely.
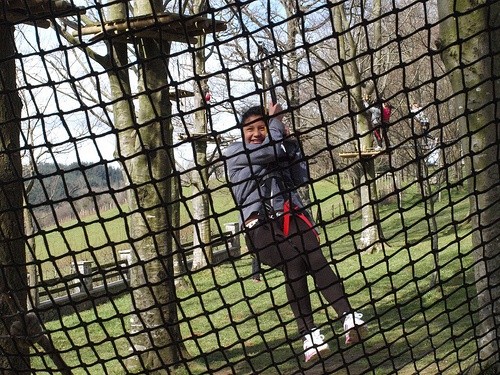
[302,328,330,363]
[342,311,368,345]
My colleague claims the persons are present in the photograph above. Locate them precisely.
[221,103,370,362]
[410,100,436,144]
[362,97,392,152]
